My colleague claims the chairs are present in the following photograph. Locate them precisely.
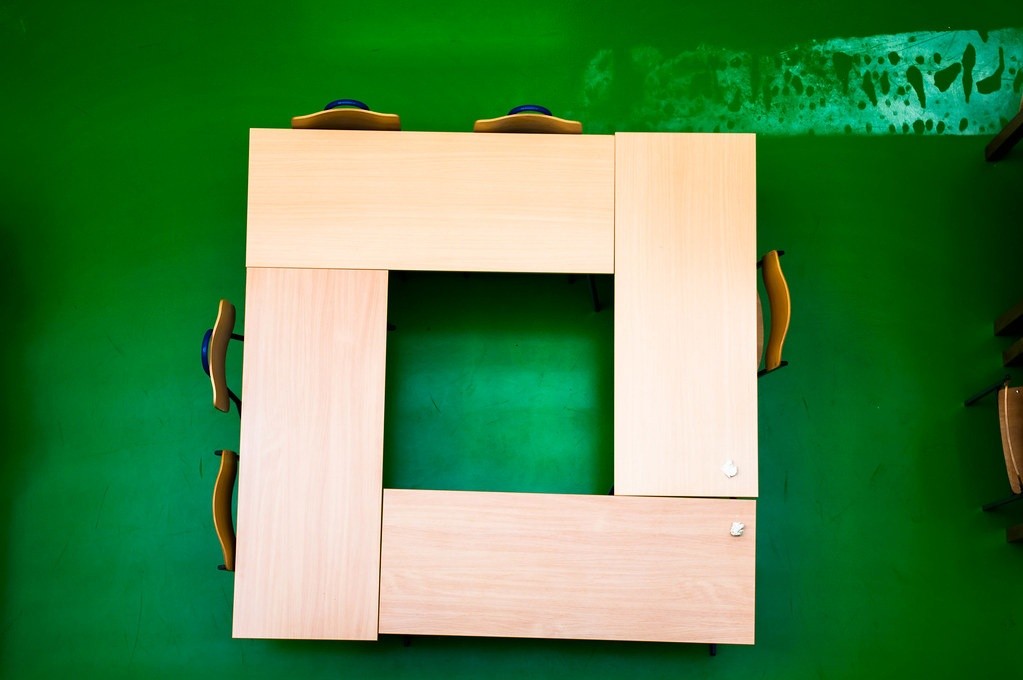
[965,374,1023,512]
[755,250,792,377]
[291,99,402,131]
[474,105,582,135]
[213,450,240,570]
[201,300,241,416]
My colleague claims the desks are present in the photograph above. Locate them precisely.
[231,128,759,645]
